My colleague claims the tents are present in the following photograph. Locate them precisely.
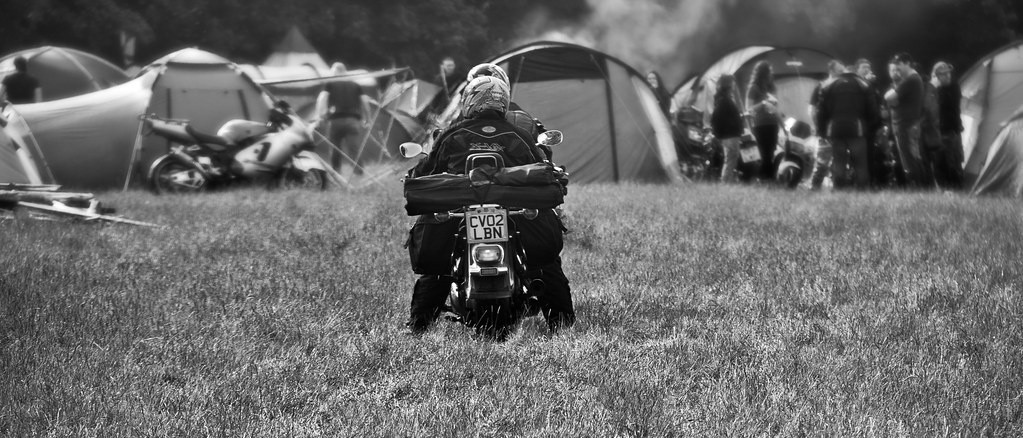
[0,27,442,195]
[954,39,1023,198]
[438,40,685,184]
[0,46,133,108]
[673,41,846,137]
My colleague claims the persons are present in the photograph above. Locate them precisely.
[805,54,968,191]
[0,56,42,104]
[401,62,576,339]
[709,73,753,183]
[433,57,464,114]
[316,62,366,179]
[745,59,783,183]
[647,71,671,111]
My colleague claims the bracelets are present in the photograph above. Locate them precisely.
[741,113,744,118]
[761,100,766,111]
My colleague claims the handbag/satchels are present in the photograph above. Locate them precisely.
[404,213,454,274]
[510,206,567,264]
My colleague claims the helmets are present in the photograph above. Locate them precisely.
[462,75,511,118]
[467,63,510,89]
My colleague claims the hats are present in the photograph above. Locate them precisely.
[888,52,911,64]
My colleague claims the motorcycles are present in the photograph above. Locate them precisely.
[138,98,328,193]
[399,129,570,344]
[669,97,818,190]
